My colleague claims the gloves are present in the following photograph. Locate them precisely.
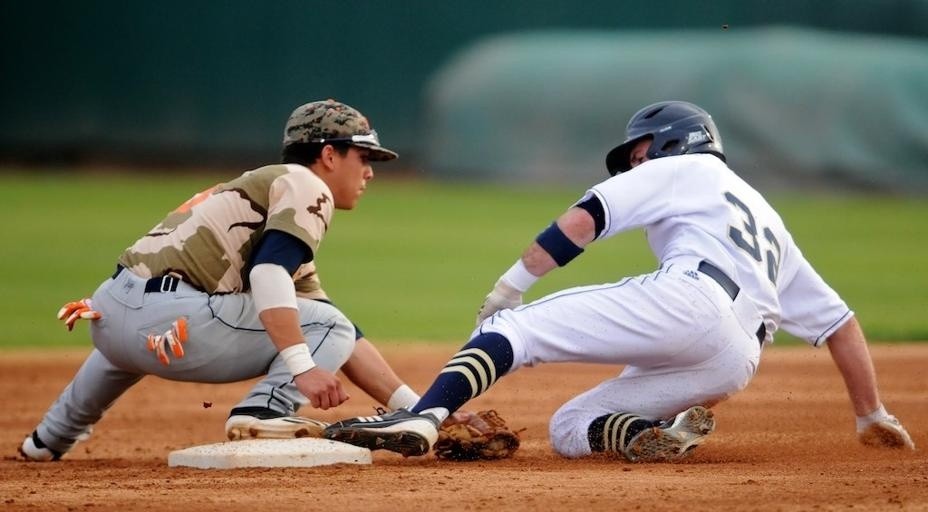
[54,295,103,331]
[474,279,524,329]
[854,406,915,456]
[148,318,191,365]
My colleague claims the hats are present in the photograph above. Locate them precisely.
[282,96,400,164]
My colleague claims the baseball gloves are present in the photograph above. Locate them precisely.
[432,409,527,459]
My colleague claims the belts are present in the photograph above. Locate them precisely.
[691,258,767,349]
[111,263,180,293]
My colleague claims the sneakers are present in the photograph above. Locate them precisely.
[623,405,717,463]
[18,431,64,462]
[321,408,439,456]
[226,410,333,442]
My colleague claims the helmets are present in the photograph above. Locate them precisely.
[605,100,726,178]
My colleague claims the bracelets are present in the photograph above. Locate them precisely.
[504,259,538,290]
[386,383,421,412]
[279,343,315,375]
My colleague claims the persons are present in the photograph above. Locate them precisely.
[18,99,517,463]
[325,101,916,464]
